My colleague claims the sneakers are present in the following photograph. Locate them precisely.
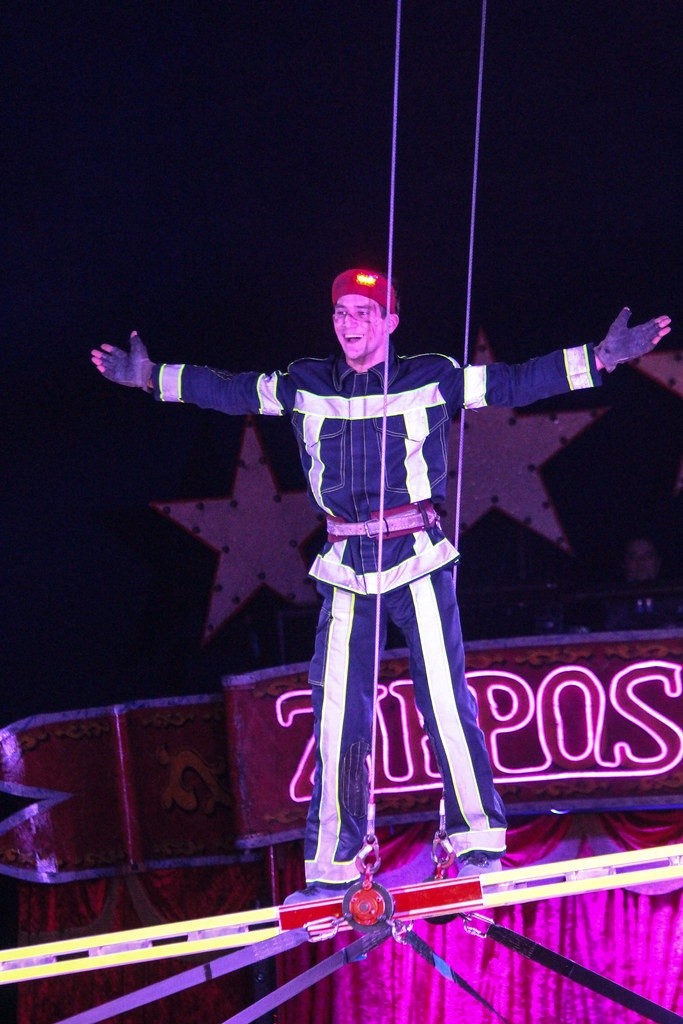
[282,884,348,906]
[458,856,503,878]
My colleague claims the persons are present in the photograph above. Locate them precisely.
[587,531,683,631]
[90,269,670,904]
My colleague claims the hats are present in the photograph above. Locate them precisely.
[331,269,397,315]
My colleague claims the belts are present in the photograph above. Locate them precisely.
[328,508,439,539]
